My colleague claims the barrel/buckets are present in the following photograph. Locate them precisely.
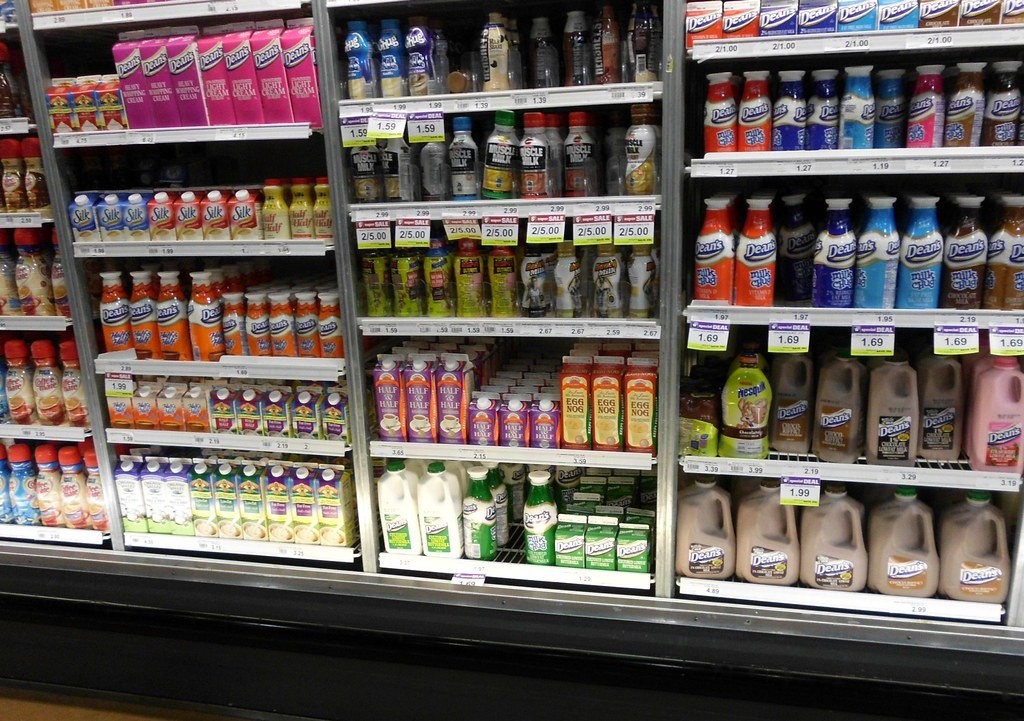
[673,470,1013,607]
[768,330,1024,474]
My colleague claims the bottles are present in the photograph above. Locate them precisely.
[461,457,586,565]
[696,188,1024,312]
[702,59,1024,159]
[345,0,662,320]
[0,40,110,533]
[718,340,772,460]
[98,262,343,364]
[260,173,335,241]
